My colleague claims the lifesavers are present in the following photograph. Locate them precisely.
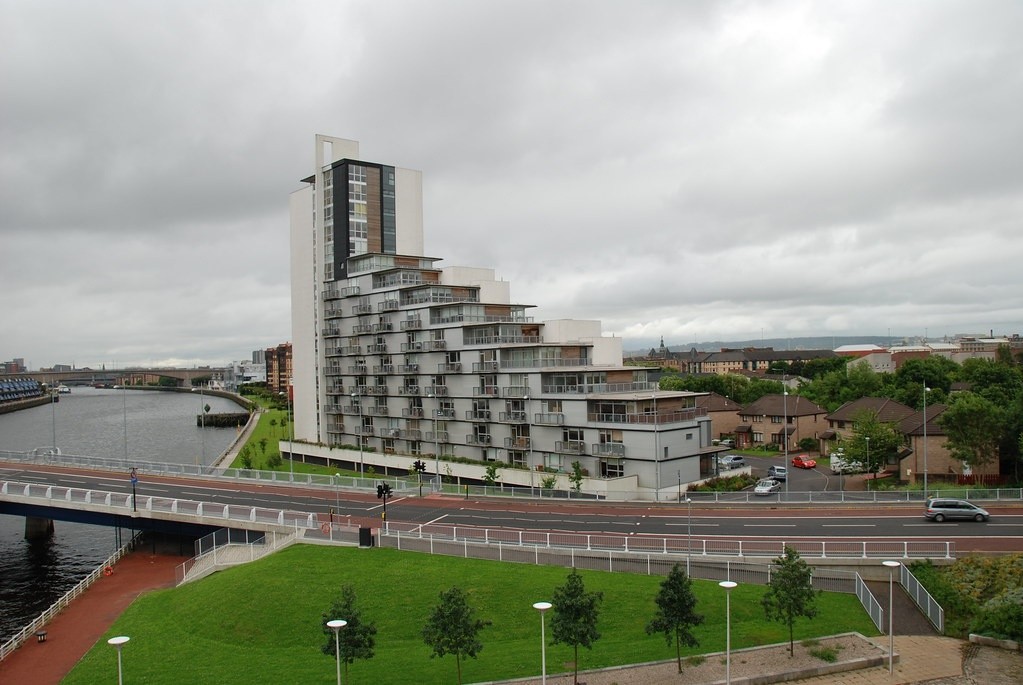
[103,566,114,576]
[320,522,330,535]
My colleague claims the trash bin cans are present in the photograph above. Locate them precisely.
[35,631,48,643]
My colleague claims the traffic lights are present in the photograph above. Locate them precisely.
[388,485,393,498]
[414,461,426,471]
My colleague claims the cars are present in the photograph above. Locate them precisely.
[830,459,871,475]
[711,440,786,496]
[924,496,989,522]
[792,454,816,469]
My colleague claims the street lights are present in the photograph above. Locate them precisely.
[523,394,534,496]
[114,383,126,466]
[326,620,348,684]
[784,392,789,501]
[42,381,55,454]
[107,636,130,685]
[882,561,901,678]
[351,392,363,485]
[428,394,439,483]
[923,387,930,500]
[719,581,737,685]
[533,602,552,685]
[191,388,205,474]
[279,391,293,480]
